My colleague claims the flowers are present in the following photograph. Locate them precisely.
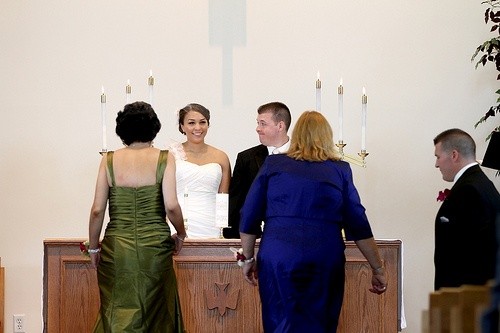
[437,188,449,202]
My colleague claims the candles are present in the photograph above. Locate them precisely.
[361,88,367,151]
[147,70,154,110]
[338,78,344,141]
[126,82,131,104]
[101,85,107,149]
[316,72,321,113]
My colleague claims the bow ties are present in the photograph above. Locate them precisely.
[436,188,449,201]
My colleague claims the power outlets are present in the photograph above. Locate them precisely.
[13,315,25,333]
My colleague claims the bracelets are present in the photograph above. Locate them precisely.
[374,266,384,272]
[234,248,255,267]
[80,241,102,257]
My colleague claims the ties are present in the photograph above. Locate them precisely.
[272,148,279,154]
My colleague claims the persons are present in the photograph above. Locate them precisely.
[238,111,387,333]
[87,102,189,333]
[228,102,295,238]
[432,128,500,291]
[161,104,233,237]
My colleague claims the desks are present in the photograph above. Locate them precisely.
[42,239,407,333]
